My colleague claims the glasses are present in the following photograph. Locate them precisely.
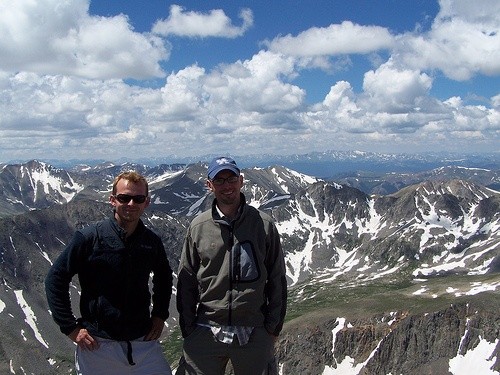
[209,175,241,185]
[113,193,147,204]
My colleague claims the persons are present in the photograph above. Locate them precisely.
[176,156,288,375]
[44,171,173,375]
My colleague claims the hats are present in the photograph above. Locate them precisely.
[207,156,241,180]
